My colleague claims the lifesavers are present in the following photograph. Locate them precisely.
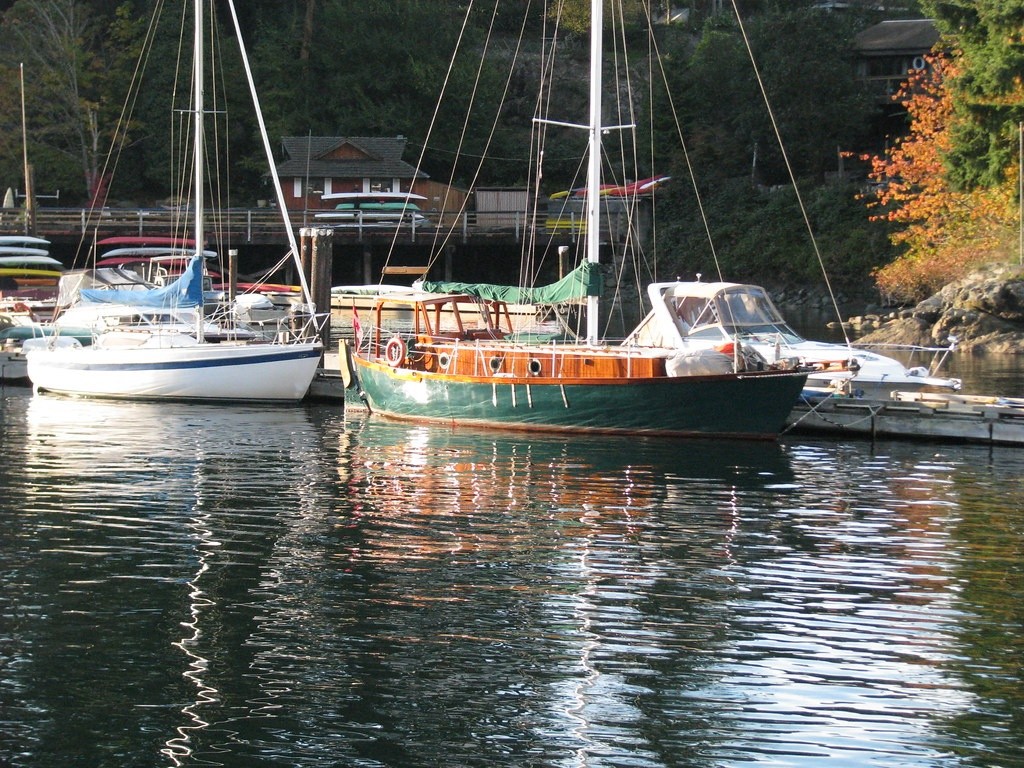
[385,337,405,368]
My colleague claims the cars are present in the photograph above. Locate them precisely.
[865,170,920,191]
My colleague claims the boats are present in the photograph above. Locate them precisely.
[619,281,962,401]
[1,312,28,380]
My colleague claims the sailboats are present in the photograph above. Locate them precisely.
[338,2,859,439]
[23,0,330,405]
